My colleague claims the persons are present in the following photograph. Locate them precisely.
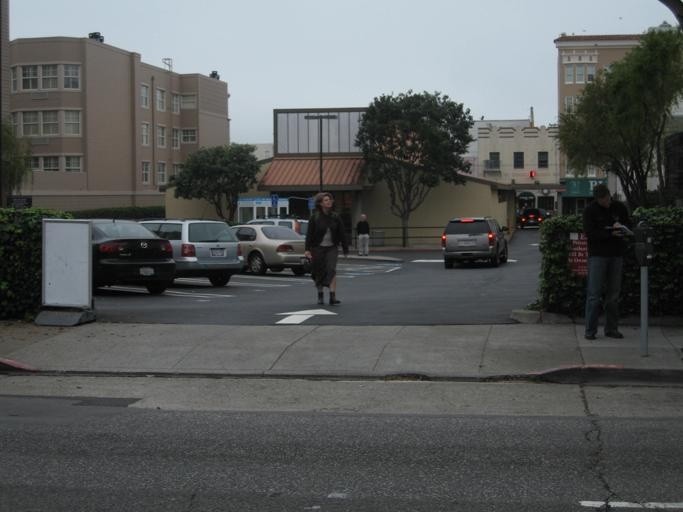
[582,183,637,340]
[353,213,370,257]
[304,191,349,305]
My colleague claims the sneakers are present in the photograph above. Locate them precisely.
[585,328,598,340]
[605,327,623,339]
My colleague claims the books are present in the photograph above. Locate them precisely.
[604,223,635,236]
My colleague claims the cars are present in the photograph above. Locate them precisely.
[86,217,177,297]
[216,224,310,274]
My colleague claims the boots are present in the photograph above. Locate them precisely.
[317,291,326,304]
[330,293,341,306]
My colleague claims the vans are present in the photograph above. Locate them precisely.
[140,219,245,289]
[245,216,314,272]
[519,208,550,229]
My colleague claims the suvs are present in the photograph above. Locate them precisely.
[440,216,507,269]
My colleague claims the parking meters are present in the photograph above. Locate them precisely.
[637,220,655,356]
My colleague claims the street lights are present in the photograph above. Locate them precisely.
[305,113,339,190]
